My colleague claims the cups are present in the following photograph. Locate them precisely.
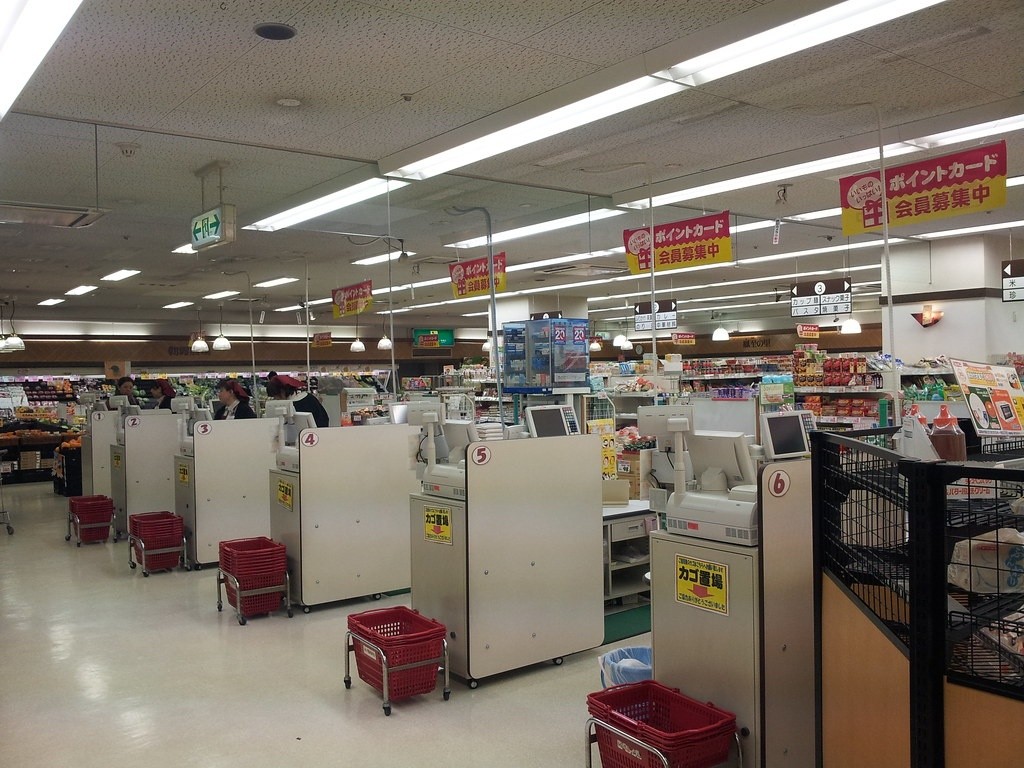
[711,388,759,399]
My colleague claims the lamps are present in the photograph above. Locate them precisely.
[911,304,945,327]
[482,314,489,351]
[0,0,1024,315]
[213,301,231,350]
[613,317,633,350]
[712,314,730,341]
[841,236,862,334]
[0,304,13,353]
[350,314,366,352]
[192,307,209,353]
[590,322,601,351]
[377,314,392,350]
[3,299,25,350]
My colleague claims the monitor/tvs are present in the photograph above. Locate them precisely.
[760,411,810,459]
[93,402,141,416]
[189,399,221,425]
[388,402,409,424]
[523,404,569,438]
[689,429,757,488]
[284,412,318,445]
[442,419,481,459]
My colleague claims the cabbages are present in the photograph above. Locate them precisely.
[132,385,149,406]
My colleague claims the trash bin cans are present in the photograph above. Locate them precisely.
[600,646,653,690]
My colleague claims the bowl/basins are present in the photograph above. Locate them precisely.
[727,360,778,373]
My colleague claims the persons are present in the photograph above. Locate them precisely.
[264,371,277,386]
[149,378,177,414]
[107,376,138,410]
[266,375,329,427]
[531,350,546,370]
[213,379,257,419]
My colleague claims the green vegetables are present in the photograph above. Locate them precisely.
[168,377,254,399]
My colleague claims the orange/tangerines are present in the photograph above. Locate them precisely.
[55,437,81,452]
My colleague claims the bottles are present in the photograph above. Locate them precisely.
[684,360,712,374]
[901,402,968,462]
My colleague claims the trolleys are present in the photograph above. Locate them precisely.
[0,448,14,535]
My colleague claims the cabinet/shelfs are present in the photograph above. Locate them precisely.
[445,349,1024,593]
[2,371,444,494]
